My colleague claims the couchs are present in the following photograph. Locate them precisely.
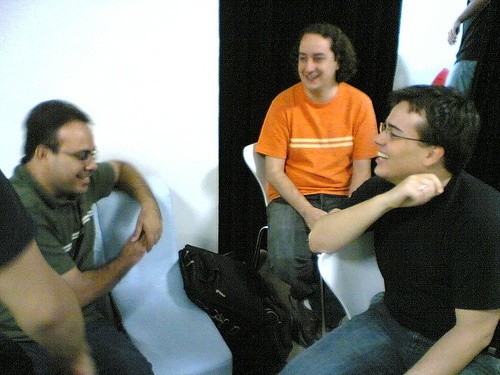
[90,175,233,375]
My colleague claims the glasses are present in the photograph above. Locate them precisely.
[60,149,99,162]
[379,122,429,142]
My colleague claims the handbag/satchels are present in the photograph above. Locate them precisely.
[179,243,292,375]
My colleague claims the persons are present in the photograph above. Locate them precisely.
[1,170,97,375]
[0,99,163,374]
[256,22,380,347]
[276,85,500,375]
[443,0,490,94]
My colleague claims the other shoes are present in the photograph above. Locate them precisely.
[295,296,320,348]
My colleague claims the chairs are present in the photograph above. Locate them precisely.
[243,142,326,339]
[317,230,385,320]
